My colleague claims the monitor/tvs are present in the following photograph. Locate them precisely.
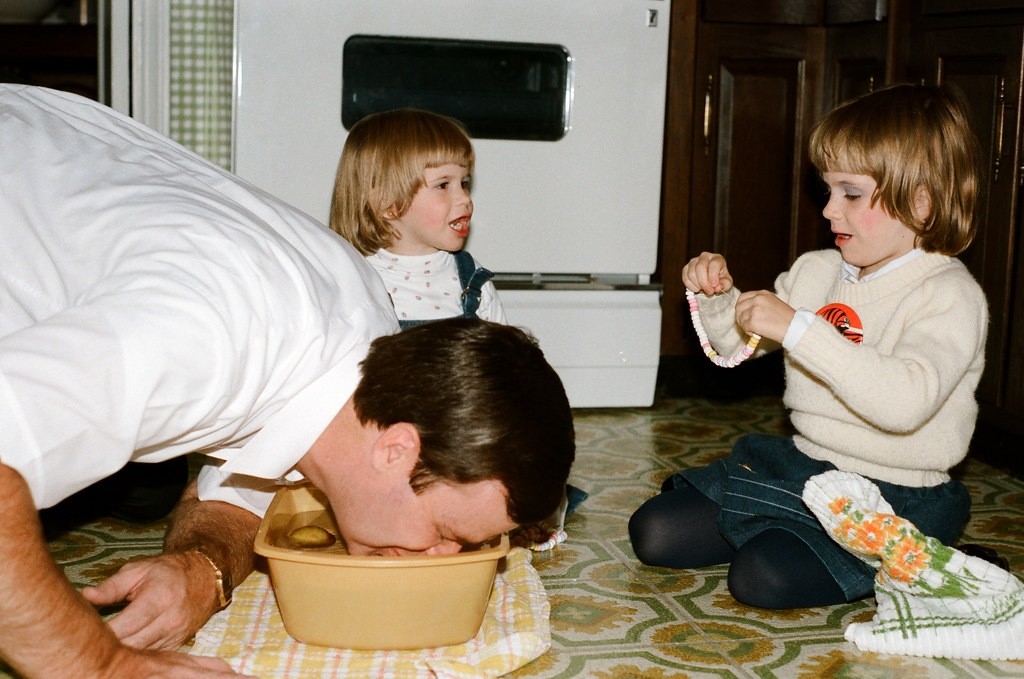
[342,34,574,142]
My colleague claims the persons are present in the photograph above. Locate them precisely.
[629,84,990,611]
[1,82,575,678]
[329,107,508,335]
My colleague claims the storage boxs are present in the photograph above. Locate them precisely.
[252,481,512,650]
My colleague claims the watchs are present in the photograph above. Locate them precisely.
[190,546,233,608]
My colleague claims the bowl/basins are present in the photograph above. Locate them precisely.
[252,481,512,648]
